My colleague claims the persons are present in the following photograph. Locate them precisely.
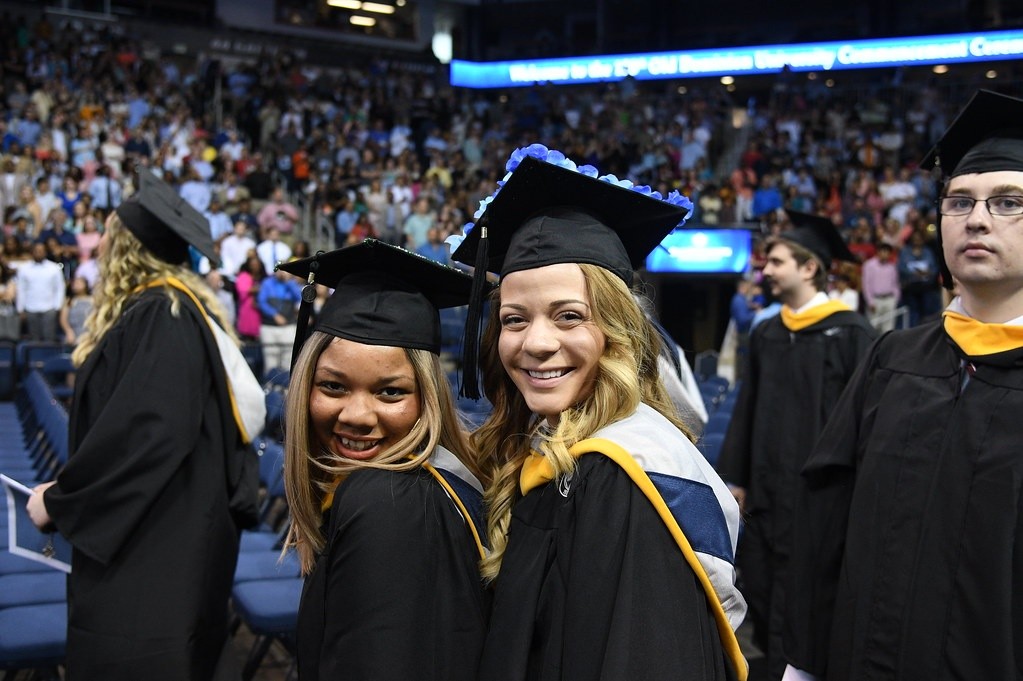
[716,206,880,654]
[273,238,501,681]
[0,15,1023,344]
[449,142,748,681]
[780,89,1023,681]
[25,163,267,681]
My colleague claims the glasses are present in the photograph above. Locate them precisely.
[933,196,1023,217]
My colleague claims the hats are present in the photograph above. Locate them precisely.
[777,208,861,292]
[916,88,1023,294]
[445,143,695,401]
[117,165,223,270]
[275,240,501,379]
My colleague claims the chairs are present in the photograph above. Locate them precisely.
[0,303,496,681]
[695,370,740,463]
